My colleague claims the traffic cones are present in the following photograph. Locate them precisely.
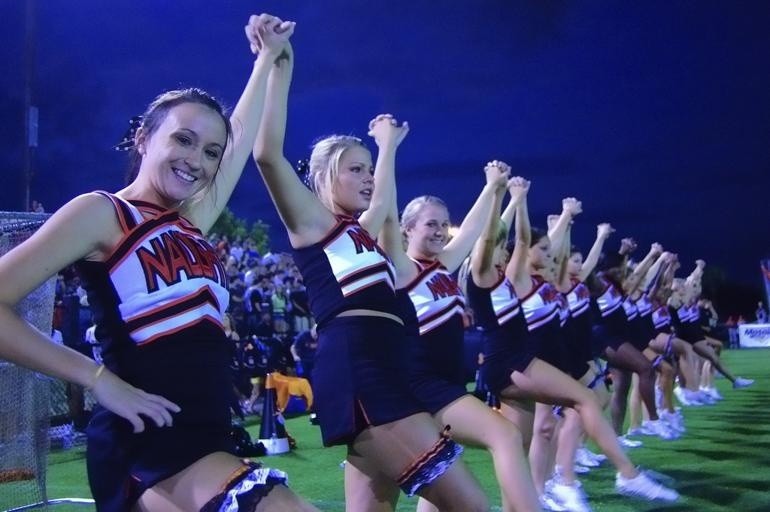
[255,371,293,456]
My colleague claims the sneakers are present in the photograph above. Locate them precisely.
[616,433,642,448]
[615,472,680,503]
[731,376,755,388]
[539,446,608,512]
[673,385,724,406]
[626,406,686,439]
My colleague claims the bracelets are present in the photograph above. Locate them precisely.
[87,363,105,391]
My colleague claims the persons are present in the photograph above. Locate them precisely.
[53,234,319,415]
[0,13,323,512]
[367,112,756,512]
[757,302,766,323]
[241,10,490,512]
